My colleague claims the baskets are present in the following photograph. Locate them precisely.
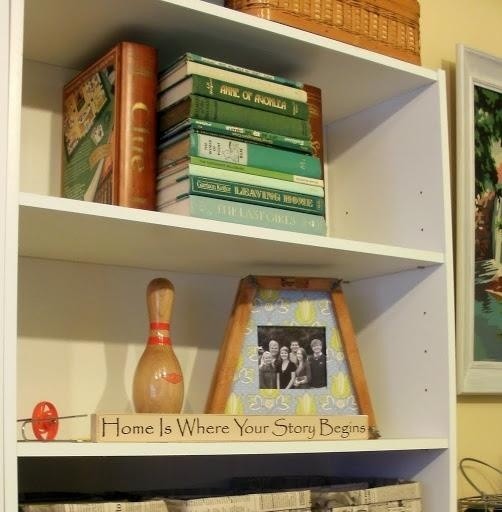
[224,0,421,67]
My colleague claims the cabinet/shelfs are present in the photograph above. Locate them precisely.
[8,0,455,511]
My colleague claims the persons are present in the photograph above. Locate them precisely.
[257,338,326,389]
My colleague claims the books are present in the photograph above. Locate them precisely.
[61,39,328,237]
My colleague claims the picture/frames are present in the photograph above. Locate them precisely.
[454,42,502,396]
[204,276,379,439]
